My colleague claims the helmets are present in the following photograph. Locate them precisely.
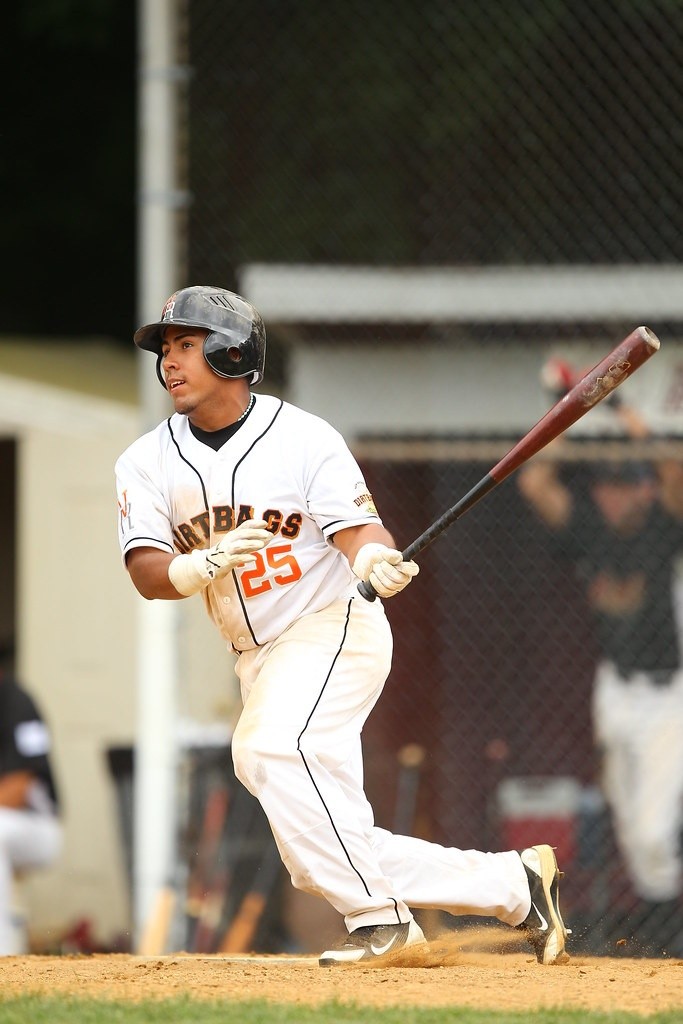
[133,286,266,387]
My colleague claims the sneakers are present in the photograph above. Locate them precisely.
[317,917,432,968]
[516,844,567,966]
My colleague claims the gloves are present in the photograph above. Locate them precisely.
[351,542,420,600]
[167,519,274,597]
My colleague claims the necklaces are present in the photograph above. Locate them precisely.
[238,392,253,423]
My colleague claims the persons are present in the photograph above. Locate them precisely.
[519,361,683,955]
[113,285,566,967]
[1,633,66,957]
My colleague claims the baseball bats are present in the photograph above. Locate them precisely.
[354,325,661,602]
